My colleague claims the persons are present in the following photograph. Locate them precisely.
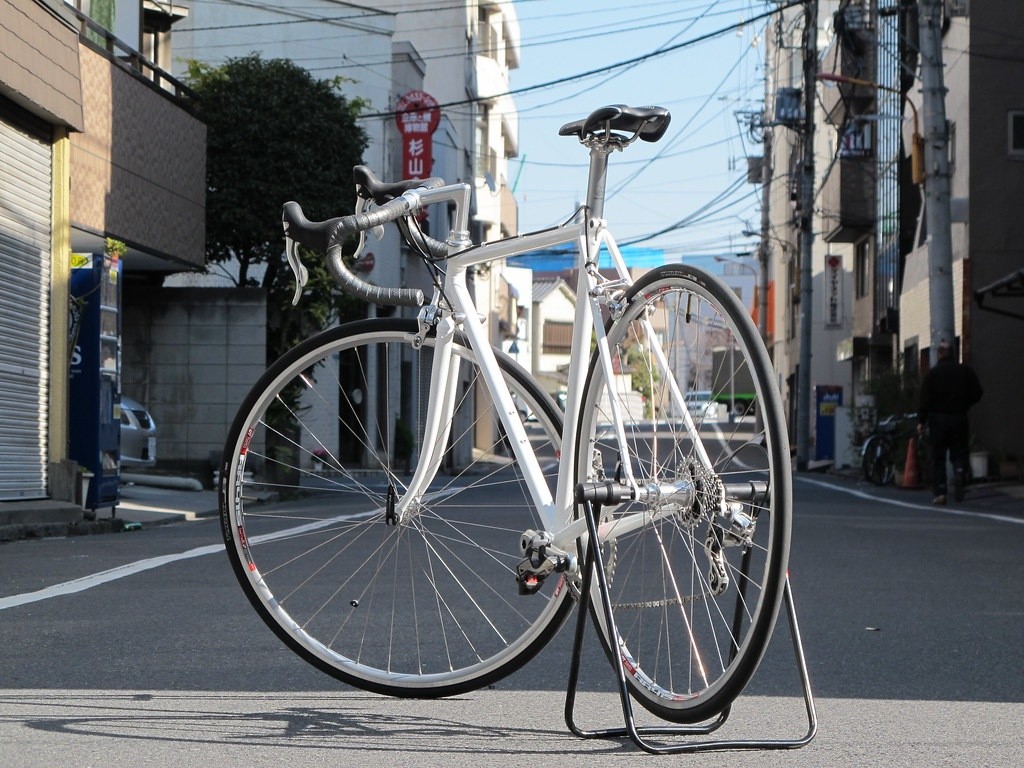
[917,342,983,504]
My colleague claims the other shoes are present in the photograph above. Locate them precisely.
[955,468,965,503]
[933,495,948,505]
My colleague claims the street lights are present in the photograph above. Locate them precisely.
[814,68,963,491]
[743,229,812,472]
[714,254,769,437]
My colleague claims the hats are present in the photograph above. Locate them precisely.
[938,340,953,349]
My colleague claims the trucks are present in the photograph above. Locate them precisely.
[710,347,756,415]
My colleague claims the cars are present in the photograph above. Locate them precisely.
[508,386,538,422]
[120,396,157,467]
[684,391,718,418]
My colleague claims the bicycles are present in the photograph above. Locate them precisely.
[862,413,918,486]
[218,103,818,756]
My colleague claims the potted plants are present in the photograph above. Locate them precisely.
[999,448,1021,482]
[967,432,991,478]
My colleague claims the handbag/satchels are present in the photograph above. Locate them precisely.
[902,431,934,489]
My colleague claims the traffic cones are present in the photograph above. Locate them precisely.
[899,437,918,488]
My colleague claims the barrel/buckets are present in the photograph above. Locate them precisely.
[969,452,989,478]
